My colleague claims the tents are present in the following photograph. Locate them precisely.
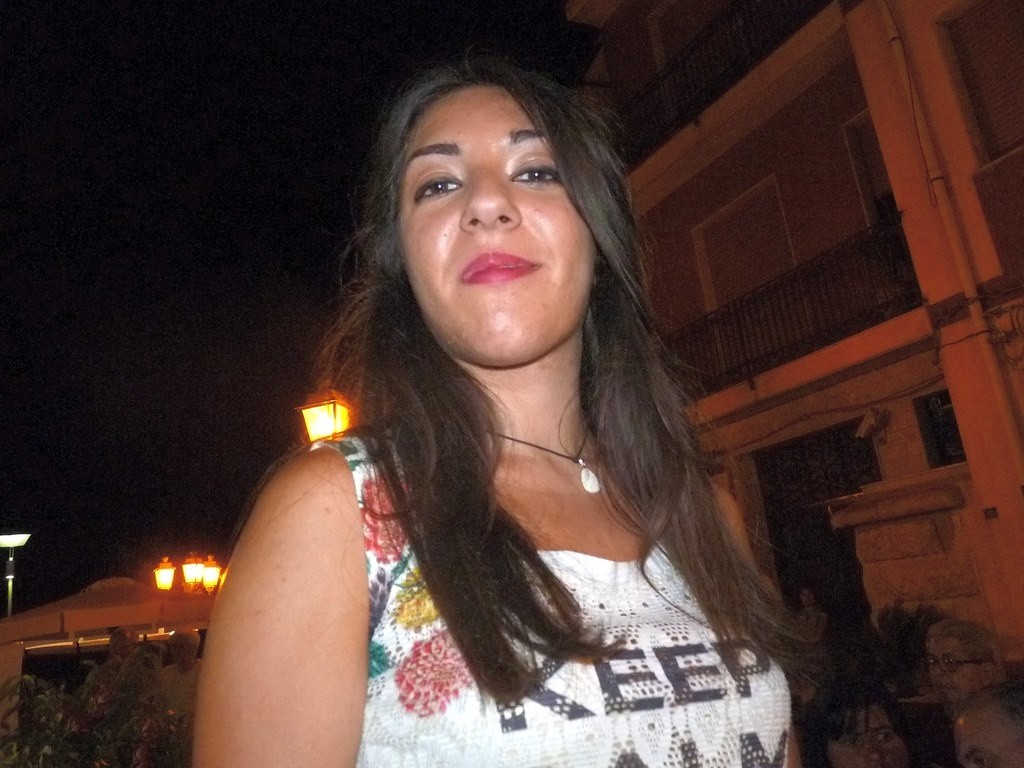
[0,576,213,740]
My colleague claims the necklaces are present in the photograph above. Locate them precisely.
[485,416,605,495]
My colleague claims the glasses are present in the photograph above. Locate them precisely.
[926,654,981,674]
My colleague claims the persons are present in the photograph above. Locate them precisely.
[190,42,802,768]
[787,580,1023,768]
[72,626,202,717]
